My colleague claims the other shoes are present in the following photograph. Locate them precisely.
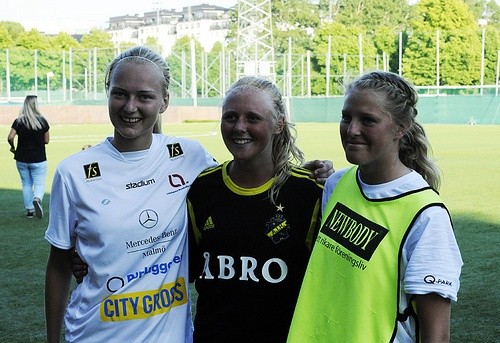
[27,213,33,218]
[33,199,43,219]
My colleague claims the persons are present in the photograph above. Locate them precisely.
[8,95,50,219]
[70,77,325,343]
[45,46,335,343]
[287,72,464,343]
